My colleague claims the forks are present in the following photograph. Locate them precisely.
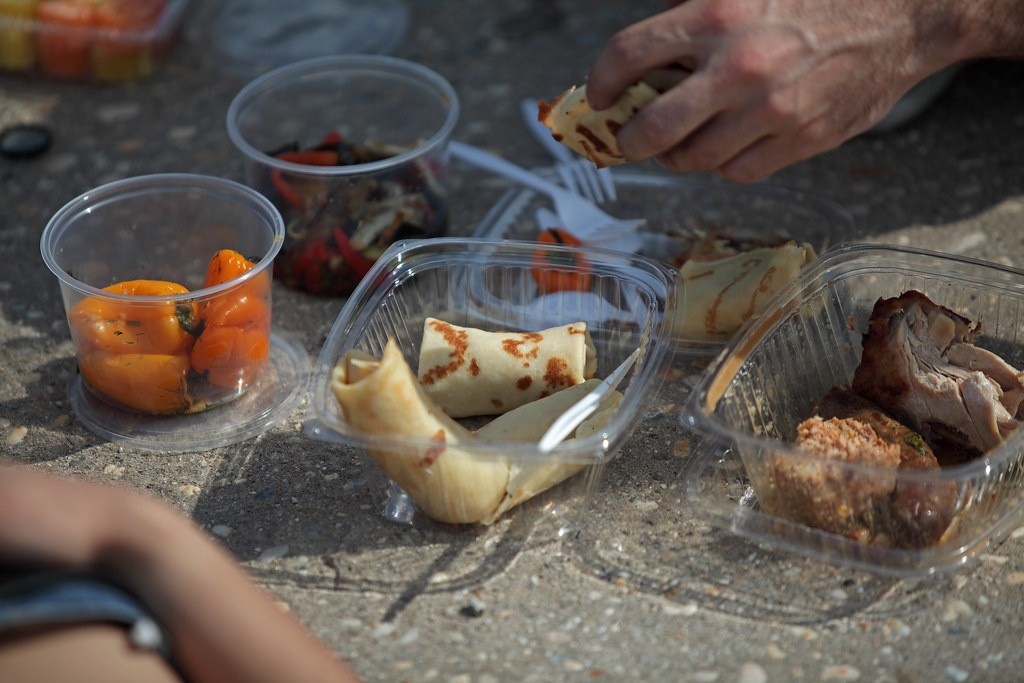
[440,137,645,243]
[522,96,620,208]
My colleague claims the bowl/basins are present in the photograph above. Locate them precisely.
[228,48,463,296]
[41,172,289,421]
[675,240,1024,555]
[304,231,687,538]
[2,0,185,86]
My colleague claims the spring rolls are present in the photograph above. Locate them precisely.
[663,240,836,344]
[535,76,710,171]
[328,312,638,528]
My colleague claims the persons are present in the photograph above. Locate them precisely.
[582,1,1023,185]
[0,448,365,683]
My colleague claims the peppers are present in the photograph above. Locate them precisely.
[69,248,271,414]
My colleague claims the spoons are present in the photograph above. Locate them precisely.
[529,289,671,330]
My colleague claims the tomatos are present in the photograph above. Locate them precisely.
[533,228,595,296]
